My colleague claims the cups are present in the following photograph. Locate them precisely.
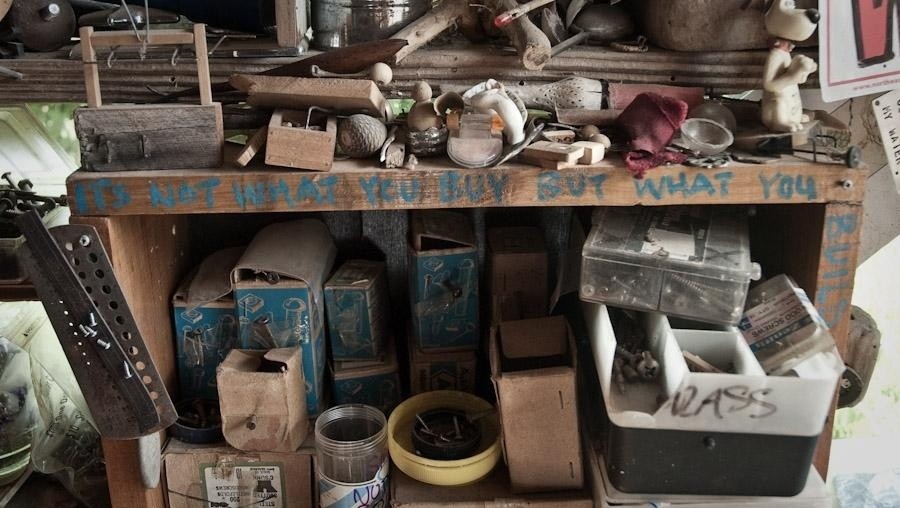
[315,403,389,508]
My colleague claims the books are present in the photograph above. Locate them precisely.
[738,268,831,378]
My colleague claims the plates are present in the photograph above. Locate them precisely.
[169,423,225,443]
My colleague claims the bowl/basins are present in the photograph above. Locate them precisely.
[681,117,734,155]
